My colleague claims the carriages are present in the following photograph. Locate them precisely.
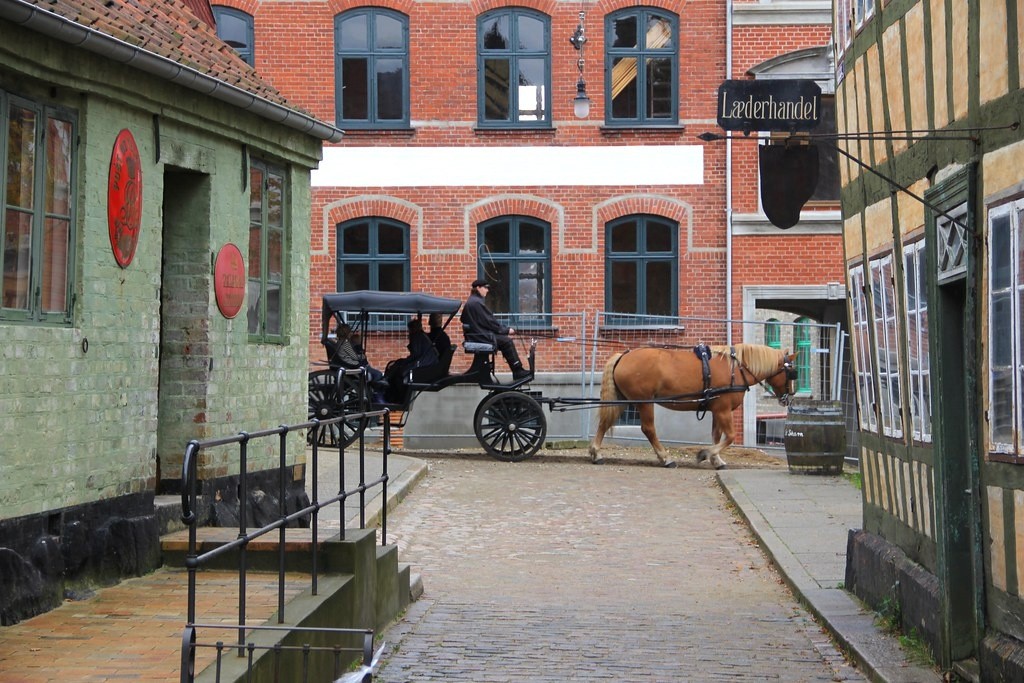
[308,291,800,469]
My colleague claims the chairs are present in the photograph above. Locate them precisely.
[326,338,359,384]
[462,324,498,354]
[408,344,457,385]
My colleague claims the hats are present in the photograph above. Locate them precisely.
[472,279,490,288]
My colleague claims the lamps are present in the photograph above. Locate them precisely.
[570,11,589,118]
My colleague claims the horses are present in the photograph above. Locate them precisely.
[589,344,800,470]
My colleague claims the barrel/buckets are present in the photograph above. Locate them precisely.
[784,398,846,476]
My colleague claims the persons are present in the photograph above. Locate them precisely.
[334,323,382,394]
[387,312,451,404]
[459,279,531,380]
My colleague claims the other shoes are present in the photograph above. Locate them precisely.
[513,367,531,381]
